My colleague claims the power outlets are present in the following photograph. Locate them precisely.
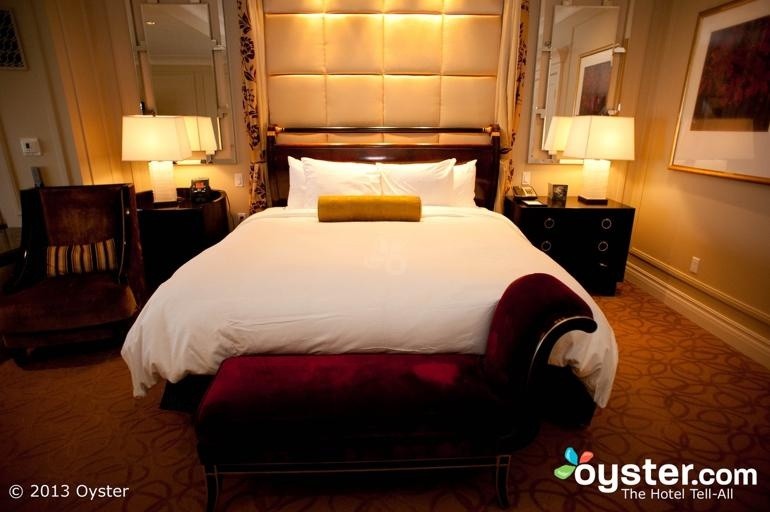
[237,213,245,226]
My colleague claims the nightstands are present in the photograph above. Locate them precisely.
[136,188,230,307]
[505,196,634,296]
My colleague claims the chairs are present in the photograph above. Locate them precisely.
[0,183,145,367]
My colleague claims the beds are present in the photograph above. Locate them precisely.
[120,124,619,430]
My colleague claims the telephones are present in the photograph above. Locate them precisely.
[513,184,537,200]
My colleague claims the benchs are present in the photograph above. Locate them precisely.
[195,273,597,512]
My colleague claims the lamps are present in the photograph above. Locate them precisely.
[563,116,635,205]
[542,116,584,164]
[174,116,222,164]
[121,115,191,203]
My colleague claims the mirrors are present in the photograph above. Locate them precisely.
[526,0,633,165]
[124,0,238,164]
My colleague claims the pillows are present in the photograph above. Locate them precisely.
[302,157,381,210]
[46,238,118,278]
[318,195,421,222]
[454,159,477,208]
[376,158,457,205]
[287,155,302,209]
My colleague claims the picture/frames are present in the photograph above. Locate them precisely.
[666,0,770,184]
[0,8,28,71]
[573,43,624,116]
